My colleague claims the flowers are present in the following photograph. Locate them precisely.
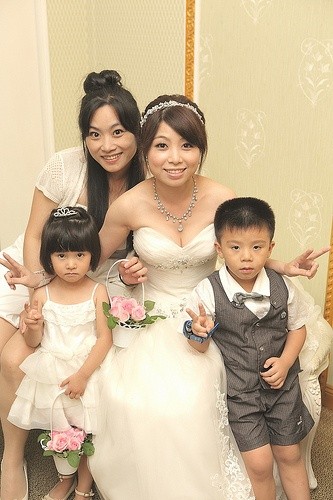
[102,295,167,329]
[38,428,95,467]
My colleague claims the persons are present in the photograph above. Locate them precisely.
[184,197,314,500]
[7,206,112,500]
[0,95,332,500]
[0,69,153,500]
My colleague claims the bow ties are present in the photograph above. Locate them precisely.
[232,292,264,309]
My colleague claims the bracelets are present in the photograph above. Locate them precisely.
[34,269,45,276]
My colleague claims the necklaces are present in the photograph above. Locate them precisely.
[153,174,198,232]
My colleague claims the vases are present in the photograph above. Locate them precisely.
[52,452,84,475]
[112,320,141,348]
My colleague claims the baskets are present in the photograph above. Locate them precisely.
[40,389,85,475]
[106,259,157,346]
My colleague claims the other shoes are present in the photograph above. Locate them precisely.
[42,473,77,500]
[0,457,28,500]
[73,486,95,500]
